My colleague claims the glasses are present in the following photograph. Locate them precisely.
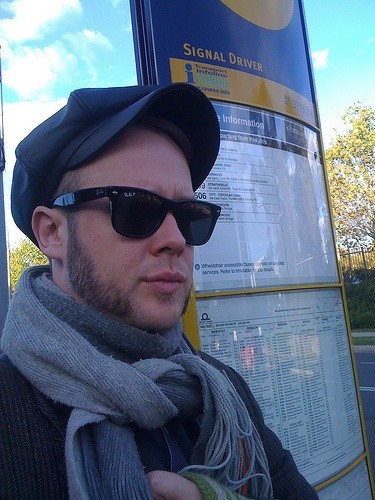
[51,186,221,247]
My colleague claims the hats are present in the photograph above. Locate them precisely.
[10,83,220,249]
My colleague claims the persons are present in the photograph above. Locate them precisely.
[0,83,318,500]
